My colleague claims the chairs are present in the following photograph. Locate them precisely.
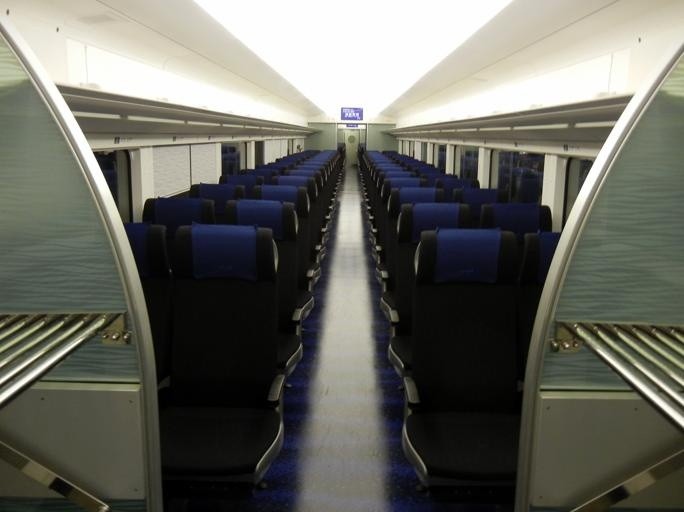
[357,140,563,494]
[122,141,344,487]
